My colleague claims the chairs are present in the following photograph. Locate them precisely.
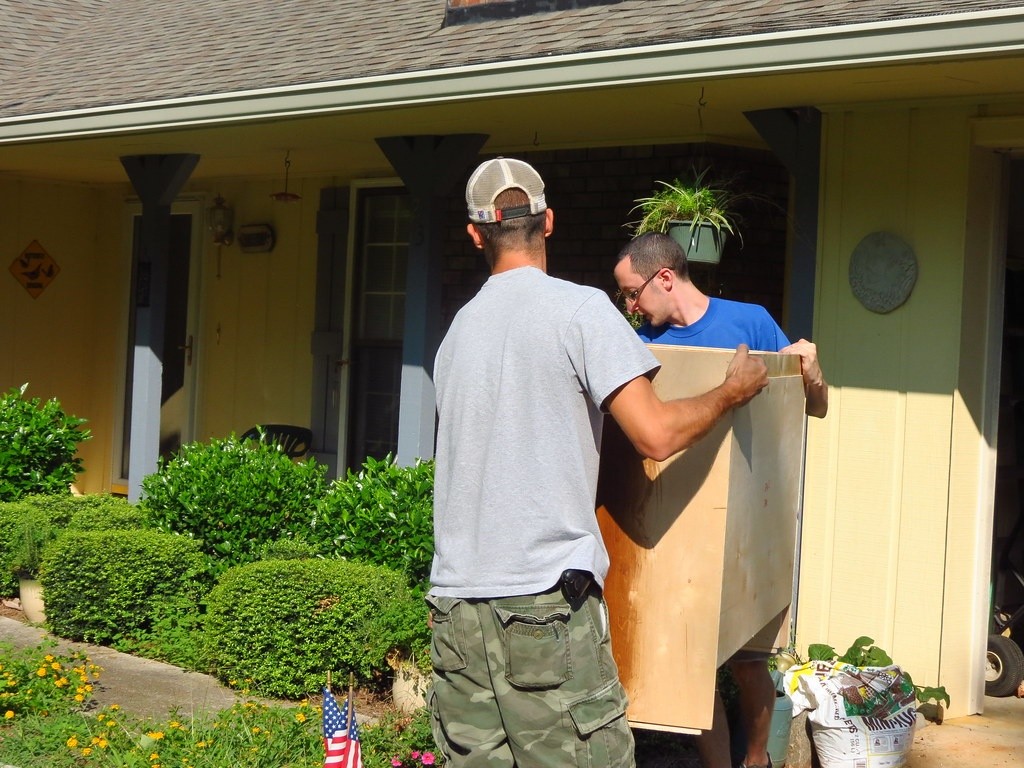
[239,425,313,461]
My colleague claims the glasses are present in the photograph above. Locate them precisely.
[623,266,676,302]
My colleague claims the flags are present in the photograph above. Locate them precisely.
[323,688,341,753]
[323,701,364,768]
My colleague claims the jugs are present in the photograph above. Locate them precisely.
[767,652,796,768]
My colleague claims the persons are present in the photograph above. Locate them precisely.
[426,156,770,768]
[615,231,827,768]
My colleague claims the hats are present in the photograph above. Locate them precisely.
[466,156,546,223]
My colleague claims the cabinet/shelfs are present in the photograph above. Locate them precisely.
[595,342,809,738]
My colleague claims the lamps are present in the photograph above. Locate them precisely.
[205,193,234,277]
[269,148,302,206]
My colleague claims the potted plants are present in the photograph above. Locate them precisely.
[390,642,435,712]
[12,525,48,623]
[615,165,810,330]
